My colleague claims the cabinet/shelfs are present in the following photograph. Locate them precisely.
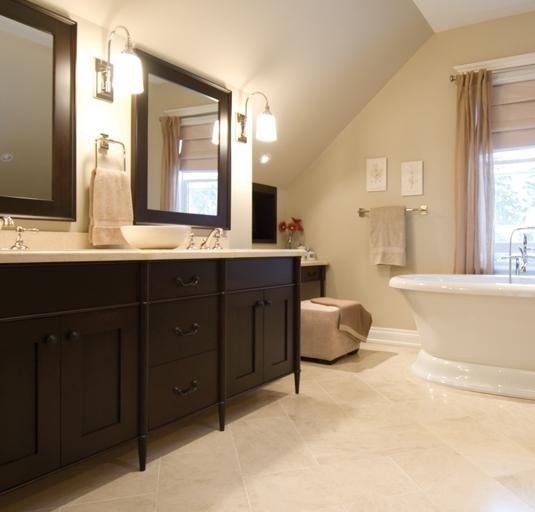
[300,260,329,297]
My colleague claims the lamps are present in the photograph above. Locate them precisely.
[95,24,145,102]
[239,90,278,145]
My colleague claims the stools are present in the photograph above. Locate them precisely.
[298,297,363,364]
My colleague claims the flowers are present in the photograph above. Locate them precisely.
[280,216,303,249]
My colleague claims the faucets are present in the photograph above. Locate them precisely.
[520,256,529,272]
[202,228,223,250]
[518,233,528,255]
[10,225,39,250]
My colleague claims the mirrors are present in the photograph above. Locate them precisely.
[0,0,81,223]
[131,48,234,232]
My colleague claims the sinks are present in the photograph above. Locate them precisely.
[119,225,192,247]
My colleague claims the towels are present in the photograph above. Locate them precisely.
[87,169,135,248]
[371,206,408,267]
[311,295,372,344]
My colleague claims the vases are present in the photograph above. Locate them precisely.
[286,230,298,249]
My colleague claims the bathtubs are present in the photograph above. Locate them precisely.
[388,274,535,403]
